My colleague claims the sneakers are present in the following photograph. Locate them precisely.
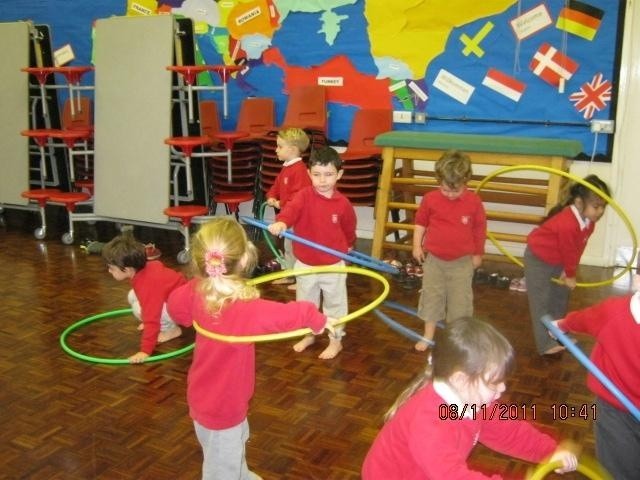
[399,263,423,290]
[254,260,281,275]
[474,268,527,292]
[144,243,162,260]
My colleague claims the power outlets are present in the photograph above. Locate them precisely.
[591,120,614,134]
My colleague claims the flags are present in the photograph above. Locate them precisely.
[554,1,605,42]
[477,69,528,111]
[567,72,613,120]
[528,42,580,89]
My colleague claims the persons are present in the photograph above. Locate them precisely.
[548,290,639,479]
[522,172,611,356]
[104,232,191,365]
[411,149,489,353]
[266,127,310,292]
[267,147,358,361]
[165,215,337,480]
[360,318,581,479]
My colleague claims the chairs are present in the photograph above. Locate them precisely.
[199,85,400,242]
